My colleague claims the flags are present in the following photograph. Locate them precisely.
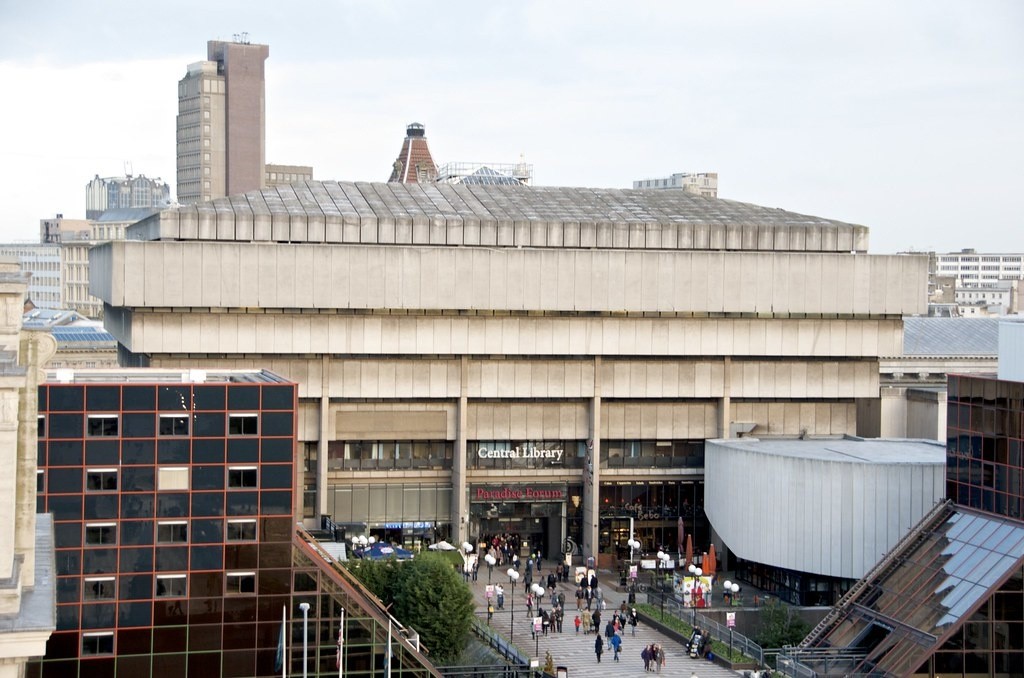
[337,619,344,673]
[384,639,393,678]
[275,622,283,672]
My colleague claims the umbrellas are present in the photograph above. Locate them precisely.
[677,517,684,558]
[685,535,692,572]
[702,545,716,575]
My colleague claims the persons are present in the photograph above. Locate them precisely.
[686,626,712,659]
[691,671,698,678]
[611,632,621,661]
[612,600,639,636]
[484,531,543,565]
[751,667,760,678]
[595,635,604,663]
[675,579,682,591]
[762,668,771,678]
[471,559,479,584]
[641,644,665,672]
[605,621,615,650]
[521,550,606,640]
[691,578,705,607]
[489,583,505,618]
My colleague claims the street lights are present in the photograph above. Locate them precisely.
[299,602,311,678]
[463,541,474,583]
[724,580,740,659]
[657,551,670,621]
[688,564,704,629]
[628,539,640,585]
[531,583,545,658]
[485,553,497,585]
[351,535,375,560]
[506,568,520,644]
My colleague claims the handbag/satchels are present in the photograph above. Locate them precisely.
[628,619,634,624]
[544,622,549,625]
[618,646,623,651]
[591,626,595,630]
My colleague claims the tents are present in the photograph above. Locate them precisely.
[353,542,414,561]
[429,541,456,551]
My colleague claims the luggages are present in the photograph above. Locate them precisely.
[649,660,657,672]
